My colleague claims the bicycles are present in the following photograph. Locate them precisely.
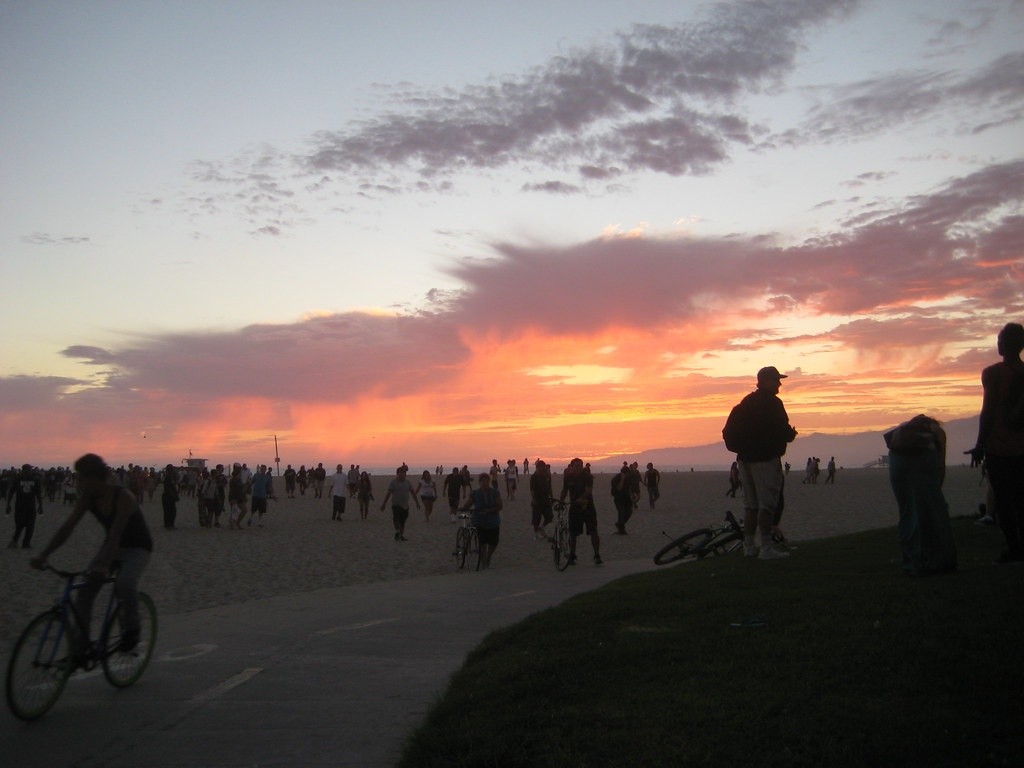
[7,559,158,721]
[549,499,574,571]
[452,508,481,572]
[654,510,744,565]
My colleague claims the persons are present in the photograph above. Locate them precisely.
[784,462,790,475]
[884,415,955,577]
[801,457,820,484]
[962,323,1024,564]
[611,462,660,536]
[30,454,154,670]
[722,367,798,561]
[826,457,837,486]
[284,458,604,570]
[0,463,276,549]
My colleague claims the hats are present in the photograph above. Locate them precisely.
[757,366,789,384]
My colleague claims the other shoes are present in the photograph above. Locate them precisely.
[120,623,142,654]
[594,554,604,566]
[394,532,400,542]
[615,522,629,535]
[400,536,407,541]
[570,556,575,565]
